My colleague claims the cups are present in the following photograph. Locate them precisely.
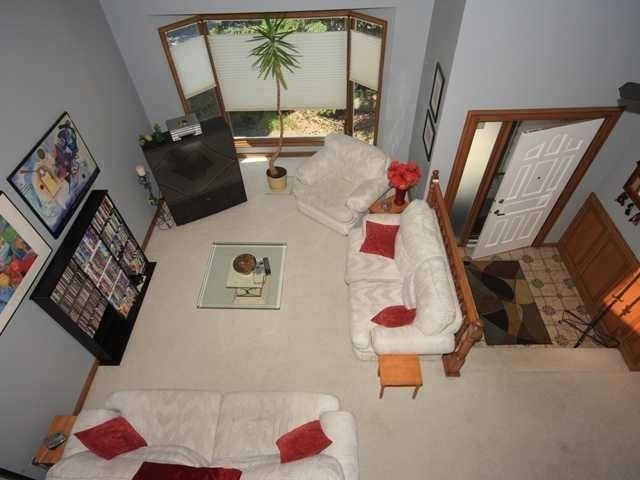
[381,200,391,208]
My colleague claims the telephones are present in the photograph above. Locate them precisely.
[44,432,67,448]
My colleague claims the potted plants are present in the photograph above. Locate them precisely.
[241,11,305,192]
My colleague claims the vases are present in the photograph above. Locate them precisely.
[392,188,407,207]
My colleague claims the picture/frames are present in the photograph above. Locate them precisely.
[427,60,446,124]
[5,110,102,240]
[420,109,437,163]
[0,188,54,336]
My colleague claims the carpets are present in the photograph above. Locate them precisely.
[462,258,553,348]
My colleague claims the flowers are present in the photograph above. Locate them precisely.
[386,160,421,191]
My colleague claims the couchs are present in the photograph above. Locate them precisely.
[42,385,363,480]
[344,170,486,379]
[292,131,390,238]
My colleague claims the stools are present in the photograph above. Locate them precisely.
[375,353,425,400]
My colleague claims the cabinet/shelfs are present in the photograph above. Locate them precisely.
[27,188,158,368]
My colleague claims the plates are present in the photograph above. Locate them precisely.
[232,254,256,273]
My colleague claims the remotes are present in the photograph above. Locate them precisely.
[263,256,272,275]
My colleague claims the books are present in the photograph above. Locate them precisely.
[49,195,147,340]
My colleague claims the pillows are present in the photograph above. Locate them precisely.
[357,220,400,260]
[369,304,417,329]
[275,419,333,465]
[71,415,148,461]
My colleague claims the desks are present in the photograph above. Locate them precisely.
[29,414,76,467]
[368,195,408,214]
